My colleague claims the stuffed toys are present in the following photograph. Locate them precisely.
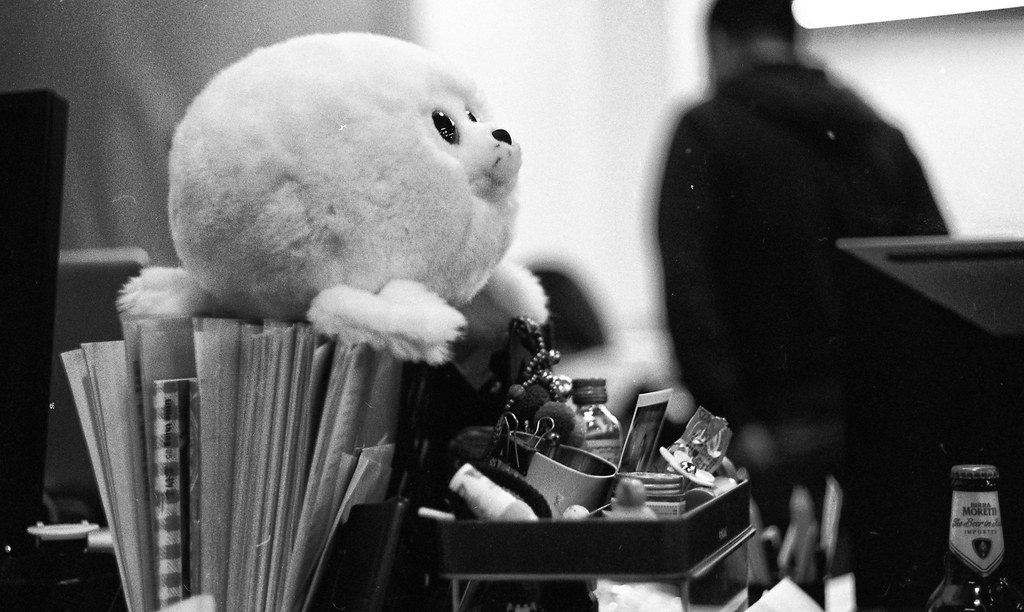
[114,28,552,360]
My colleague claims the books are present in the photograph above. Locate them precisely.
[57,317,400,612]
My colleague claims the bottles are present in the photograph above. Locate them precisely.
[926,462,1024,611]
[567,377,623,470]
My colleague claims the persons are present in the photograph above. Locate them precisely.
[656,0,954,612]
